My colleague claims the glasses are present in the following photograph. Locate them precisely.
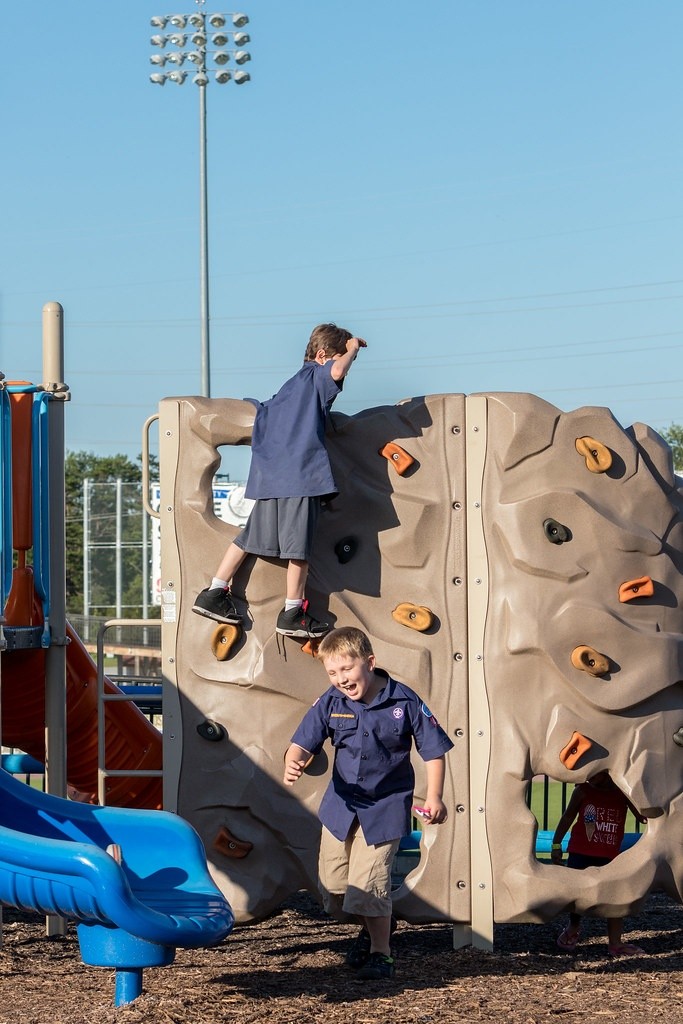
[413,806,446,825]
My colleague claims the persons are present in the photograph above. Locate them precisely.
[551,769,645,953]
[192,323,367,637]
[284,628,454,983]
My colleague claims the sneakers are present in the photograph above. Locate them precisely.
[275,598,330,638]
[346,927,372,969]
[353,952,394,984]
[192,585,243,625]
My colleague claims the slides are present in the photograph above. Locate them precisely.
[0,568,165,812]
[0,764,237,950]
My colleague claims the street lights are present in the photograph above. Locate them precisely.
[148,1,253,399]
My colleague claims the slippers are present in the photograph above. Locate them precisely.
[608,943,646,956]
[558,928,578,948]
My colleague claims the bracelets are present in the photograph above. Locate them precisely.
[552,844,562,850]
